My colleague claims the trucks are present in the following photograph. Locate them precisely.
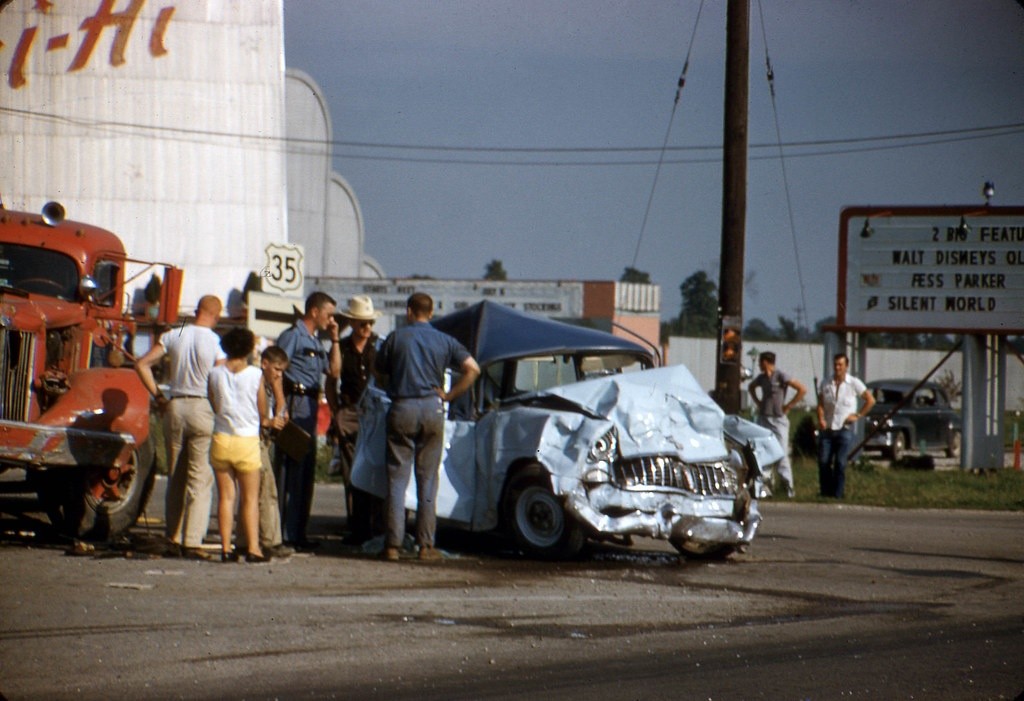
[1,200,184,552]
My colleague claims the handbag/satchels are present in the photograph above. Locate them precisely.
[277,420,314,462]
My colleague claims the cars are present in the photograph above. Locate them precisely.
[347,297,795,563]
[800,379,964,462]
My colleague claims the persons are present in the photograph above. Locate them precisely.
[137,291,480,564]
[748,352,806,498]
[816,353,877,500]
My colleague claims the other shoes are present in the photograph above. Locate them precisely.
[418,547,443,561]
[386,546,400,560]
[169,543,182,556]
[787,487,796,500]
[245,553,270,562]
[180,546,211,560]
[284,540,320,553]
[222,551,239,562]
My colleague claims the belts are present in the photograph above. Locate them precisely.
[171,395,200,399]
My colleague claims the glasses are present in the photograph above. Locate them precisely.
[357,320,376,328]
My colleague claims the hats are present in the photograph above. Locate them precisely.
[339,294,384,320]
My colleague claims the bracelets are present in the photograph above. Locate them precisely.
[330,336,340,344]
[154,393,164,402]
[855,413,863,420]
